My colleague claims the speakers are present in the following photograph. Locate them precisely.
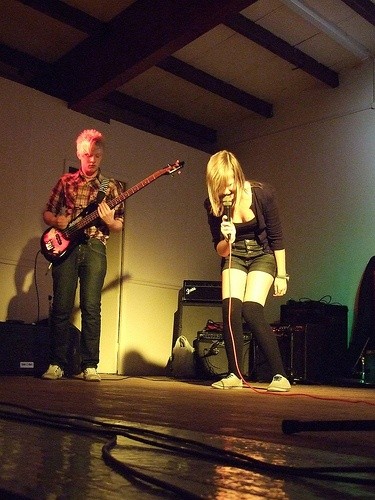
[279,302,348,383]
[28,316,84,374]
[173,303,249,378]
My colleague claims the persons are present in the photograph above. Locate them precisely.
[38,130,124,382]
[203,150,292,393]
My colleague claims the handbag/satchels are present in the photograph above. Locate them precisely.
[171,335,196,377]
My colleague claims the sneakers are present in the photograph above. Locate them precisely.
[268,375,292,391]
[84,367,101,381]
[211,373,242,390]
[42,364,64,380]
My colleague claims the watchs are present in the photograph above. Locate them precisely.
[276,273,290,282]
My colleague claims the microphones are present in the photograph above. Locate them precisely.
[223,194,233,241]
[211,342,219,355]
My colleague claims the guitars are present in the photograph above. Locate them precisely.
[39,159,185,263]
